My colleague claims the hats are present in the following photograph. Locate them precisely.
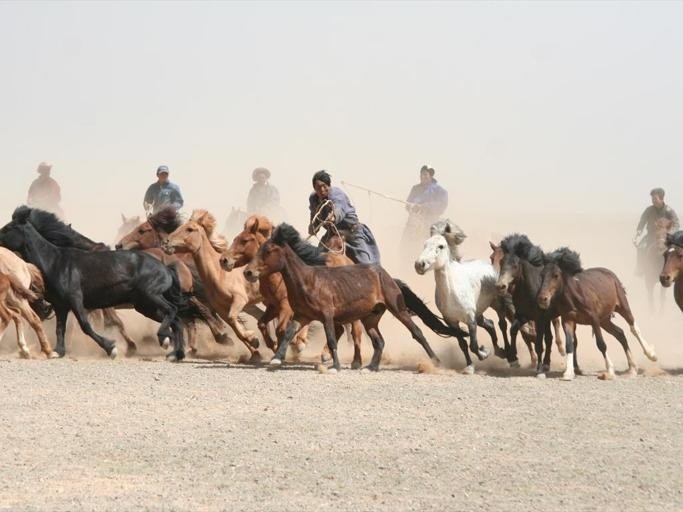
[158,166,168,176]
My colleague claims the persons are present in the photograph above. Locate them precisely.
[406,166,448,230]
[142,166,184,221]
[308,170,379,266]
[25,162,61,211]
[632,188,680,276]
[246,167,280,225]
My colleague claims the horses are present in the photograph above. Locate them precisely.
[635,211,683,312]
[4,206,658,379]
